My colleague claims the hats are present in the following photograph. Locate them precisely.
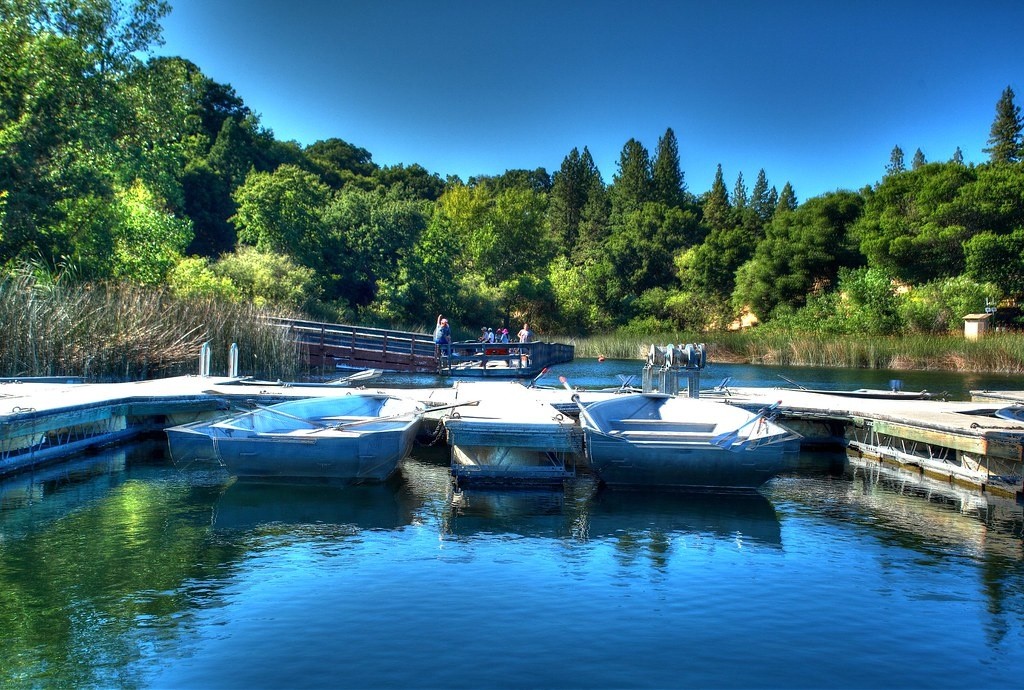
[481,327,487,331]
[441,319,448,323]
[502,329,508,334]
[497,328,501,331]
[488,328,492,331]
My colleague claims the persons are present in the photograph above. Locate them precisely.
[483,327,494,343]
[479,327,488,341]
[499,329,510,343]
[495,328,502,343]
[435,315,452,355]
[518,322,533,354]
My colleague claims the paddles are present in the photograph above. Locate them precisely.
[200,388,483,434]
[614,373,638,395]
[709,399,791,454]
[558,375,606,433]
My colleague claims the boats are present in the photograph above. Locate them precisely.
[579,389,805,490]
[163,392,431,486]
